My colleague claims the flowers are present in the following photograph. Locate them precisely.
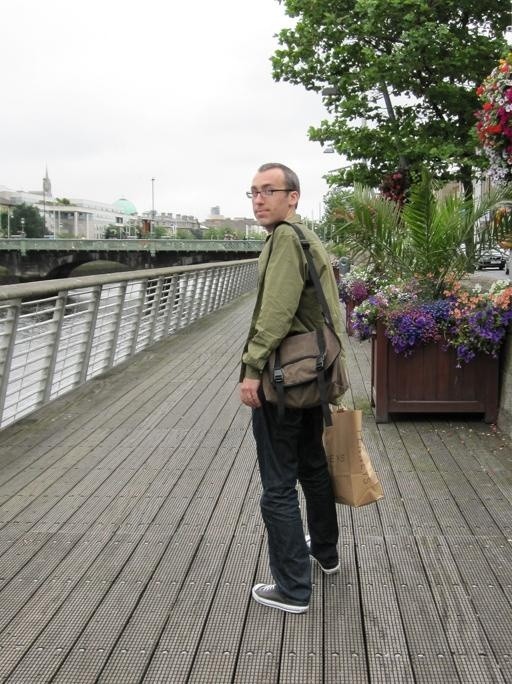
[332,257,510,372]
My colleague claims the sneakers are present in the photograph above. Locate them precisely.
[304,534,341,574]
[251,582,310,614]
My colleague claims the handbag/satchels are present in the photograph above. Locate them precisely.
[261,325,350,410]
[322,406,385,507]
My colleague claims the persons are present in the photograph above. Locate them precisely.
[236,161,352,617]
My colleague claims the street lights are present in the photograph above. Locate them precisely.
[20,217,25,238]
[151,178,157,220]
[7,209,14,238]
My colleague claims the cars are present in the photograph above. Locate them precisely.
[457,244,512,279]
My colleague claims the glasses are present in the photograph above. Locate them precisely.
[246,188,294,198]
